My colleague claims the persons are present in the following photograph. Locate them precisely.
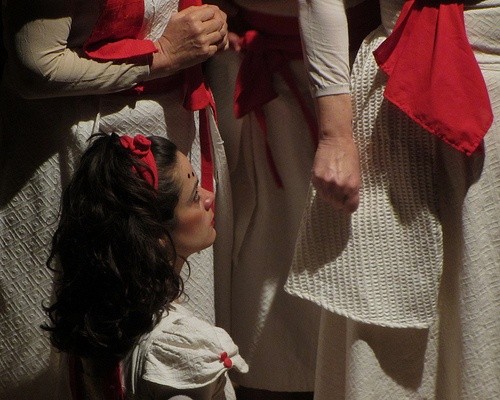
[292,0,499,400]
[0,1,254,400]
[37,132,252,400]
